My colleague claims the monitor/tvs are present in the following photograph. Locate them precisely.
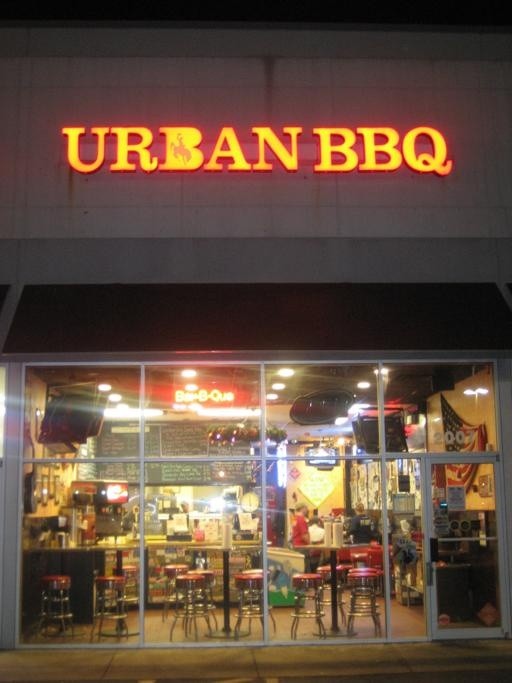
[38,395,108,442]
[304,446,340,471]
[352,415,406,461]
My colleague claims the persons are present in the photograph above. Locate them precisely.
[341,500,374,544]
[288,501,312,573]
[179,500,190,525]
[308,507,325,530]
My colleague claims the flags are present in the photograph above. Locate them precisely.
[438,393,489,497]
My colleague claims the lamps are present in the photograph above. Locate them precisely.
[241,479,259,512]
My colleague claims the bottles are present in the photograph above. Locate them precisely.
[324,521,343,547]
[57,507,77,549]
[224,524,232,548]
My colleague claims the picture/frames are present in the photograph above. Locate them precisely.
[25,467,60,514]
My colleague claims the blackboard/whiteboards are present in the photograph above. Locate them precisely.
[96,418,254,486]
